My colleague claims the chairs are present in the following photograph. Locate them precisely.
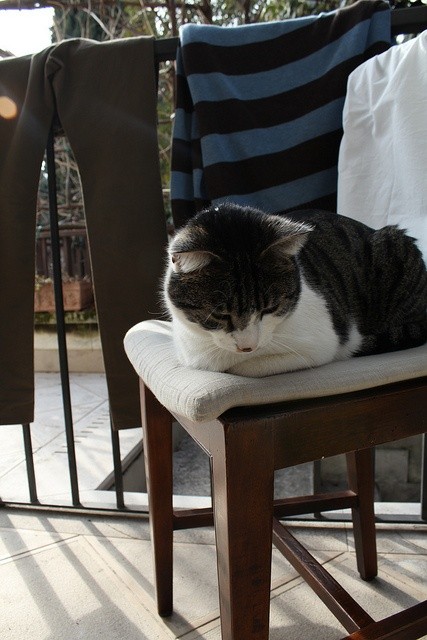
[120,33,426,639]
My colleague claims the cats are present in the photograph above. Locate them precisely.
[163,202,427,378]
